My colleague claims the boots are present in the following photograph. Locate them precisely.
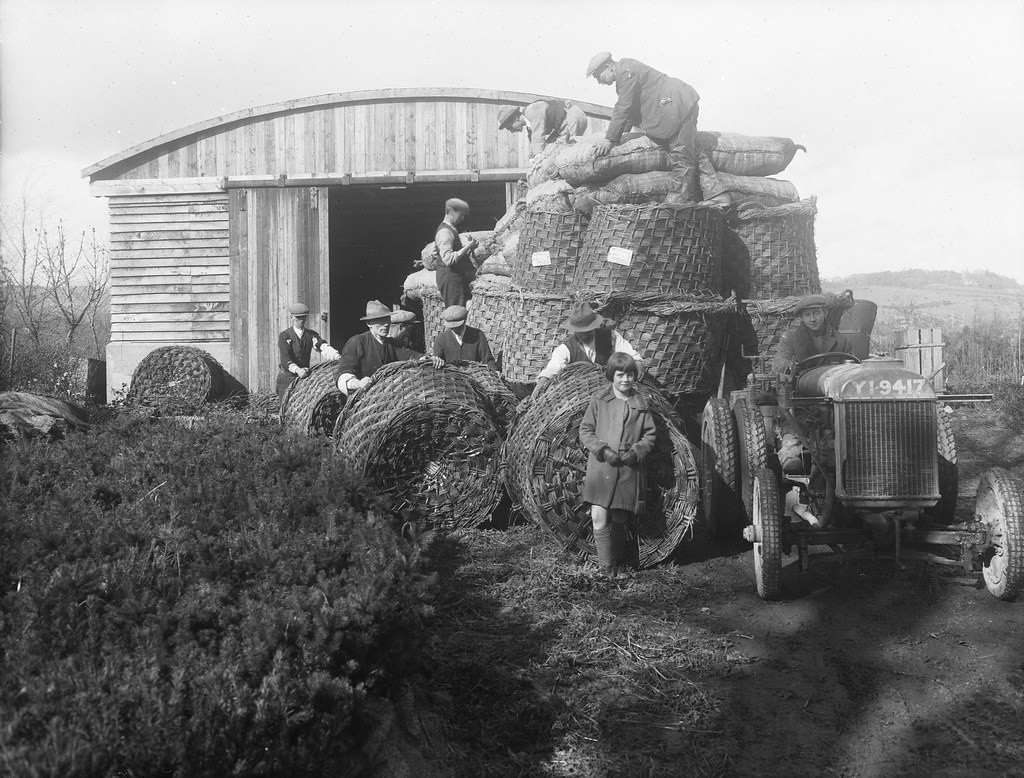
[591,520,627,574]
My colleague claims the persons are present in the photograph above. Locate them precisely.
[336,300,445,407]
[771,293,854,476]
[586,51,702,208]
[515,300,645,413]
[387,309,421,341]
[496,97,589,165]
[577,351,657,581]
[275,303,341,403]
[433,304,502,377]
[434,197,481,307]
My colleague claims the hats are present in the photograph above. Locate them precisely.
[445,198,470,217]
[558,301,604,333]
[585,51,612,78]
[497,105,520,130]
[360,300,397,320]
[288,303,310,317]
[440,305,468,328]
[794,295,827,316]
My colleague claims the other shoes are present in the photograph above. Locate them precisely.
[698,195,731,207]
[782,457,803,476]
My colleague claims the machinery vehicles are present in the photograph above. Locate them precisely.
[699,342,1024,598]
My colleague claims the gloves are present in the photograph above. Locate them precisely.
[602,448,623,468]
[622,450,638,467]
